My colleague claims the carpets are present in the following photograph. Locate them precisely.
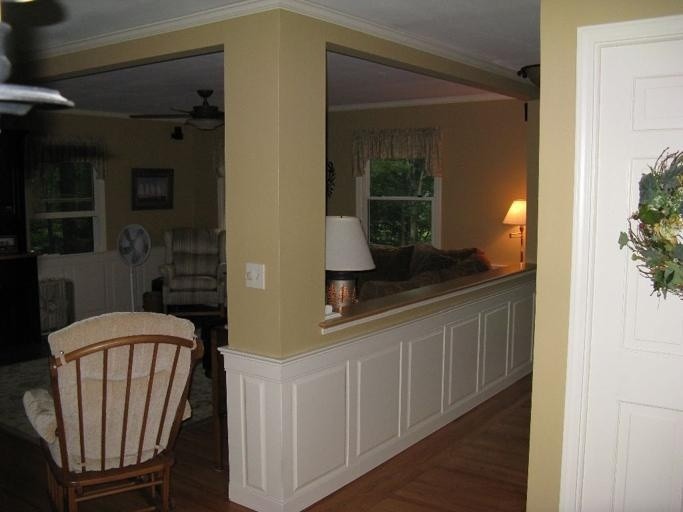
[0,318,226,456]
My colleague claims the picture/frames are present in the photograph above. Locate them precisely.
[129,166,174,212]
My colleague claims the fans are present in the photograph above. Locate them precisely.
[128,85,226,120]
[114,221,153,313]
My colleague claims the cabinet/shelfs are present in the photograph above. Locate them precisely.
[0,116,46,359]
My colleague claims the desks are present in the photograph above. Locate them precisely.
[204,322,230,474]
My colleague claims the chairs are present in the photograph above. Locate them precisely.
[20,307,205,511]
[157,223,228,320]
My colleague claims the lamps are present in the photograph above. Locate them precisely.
[186,117,223,132]
[501,196,531,265]
[517,61,540,90]
[325,214,378,312]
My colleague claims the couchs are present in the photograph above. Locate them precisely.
[356,237,492,302]
